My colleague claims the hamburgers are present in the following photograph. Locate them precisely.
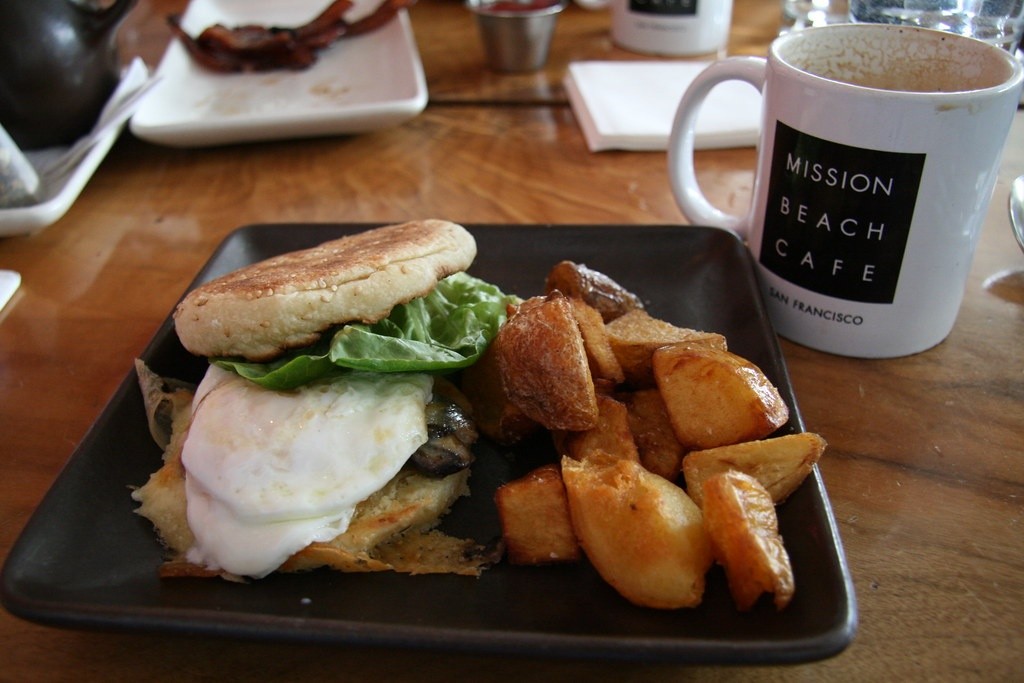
[135,217,523,579]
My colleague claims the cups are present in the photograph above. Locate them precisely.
[845,0,1024,59]
[573,0,736,57]
[464,0,570,74]
[666,21,1024,361]
[774,0,857,38]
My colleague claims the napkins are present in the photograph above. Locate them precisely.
[563,56,765,153]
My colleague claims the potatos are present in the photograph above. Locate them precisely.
[459,260,826,611]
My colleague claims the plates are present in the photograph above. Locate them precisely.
[0,218,862,666]
[0,61,154,238]
[1007,174,1024,254]
[126,0,429,148]
